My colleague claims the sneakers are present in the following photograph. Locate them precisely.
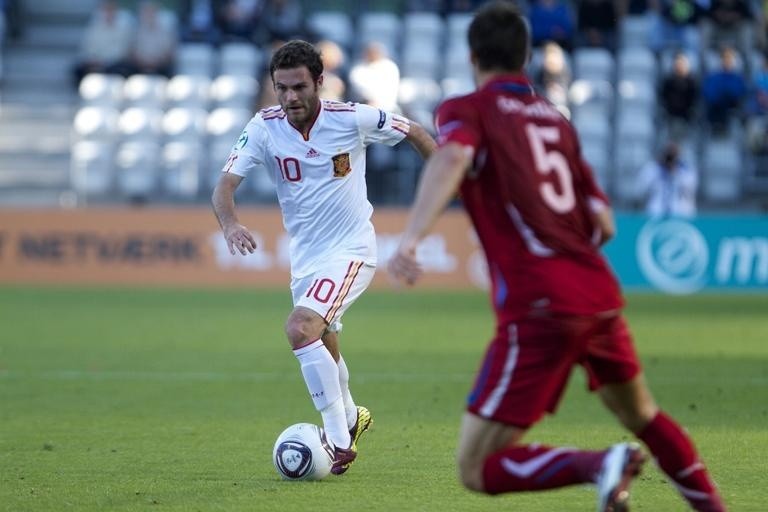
[325,406,371,474]
[597,442,643,512]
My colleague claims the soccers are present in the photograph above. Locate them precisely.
[272,422,335,480]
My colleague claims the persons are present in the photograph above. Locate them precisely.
[390,0,726,511]
[209,41,442,473]
